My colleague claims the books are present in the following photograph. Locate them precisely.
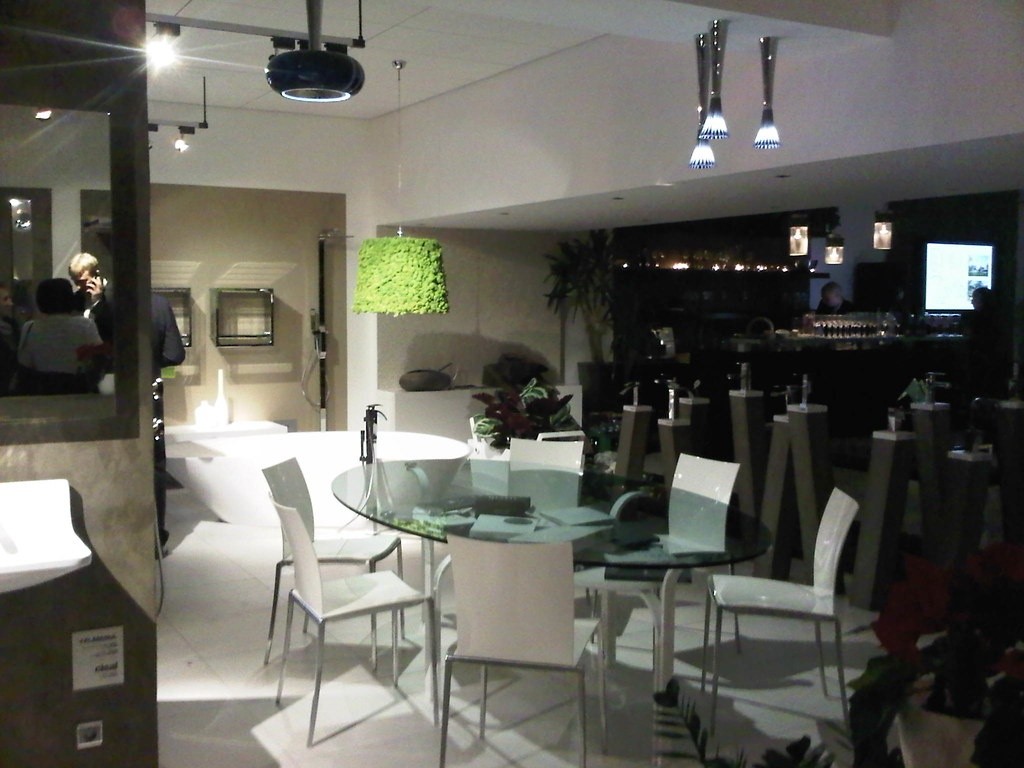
[423,515,478,532]
[469,508,618,552]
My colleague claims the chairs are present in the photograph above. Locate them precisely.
[260,423,861,768]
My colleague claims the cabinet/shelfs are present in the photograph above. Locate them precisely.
[381,384,582,464]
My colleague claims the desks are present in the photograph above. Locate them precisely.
[331,459,771,768]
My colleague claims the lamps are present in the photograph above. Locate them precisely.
[699,20,729,140]
[825,225,844,265]
[789,210,810,255]
[873,208,894,250]
[688,33,716,168]
[352,59,450,315]
[755,35,780,149]
[148,0,366,152]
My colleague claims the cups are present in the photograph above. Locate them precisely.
[802,311,964,338]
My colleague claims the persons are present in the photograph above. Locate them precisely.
[745,316,773,336]
[0,280,41,396]
[16,277,104,395]
[69,252,112,344]
[967,287,1014,398]
[96,291,186,561]
[816,283,856,314]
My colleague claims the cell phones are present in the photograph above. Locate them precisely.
[92,273,98,285]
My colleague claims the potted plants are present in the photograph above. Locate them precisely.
[543,226,658,435]
